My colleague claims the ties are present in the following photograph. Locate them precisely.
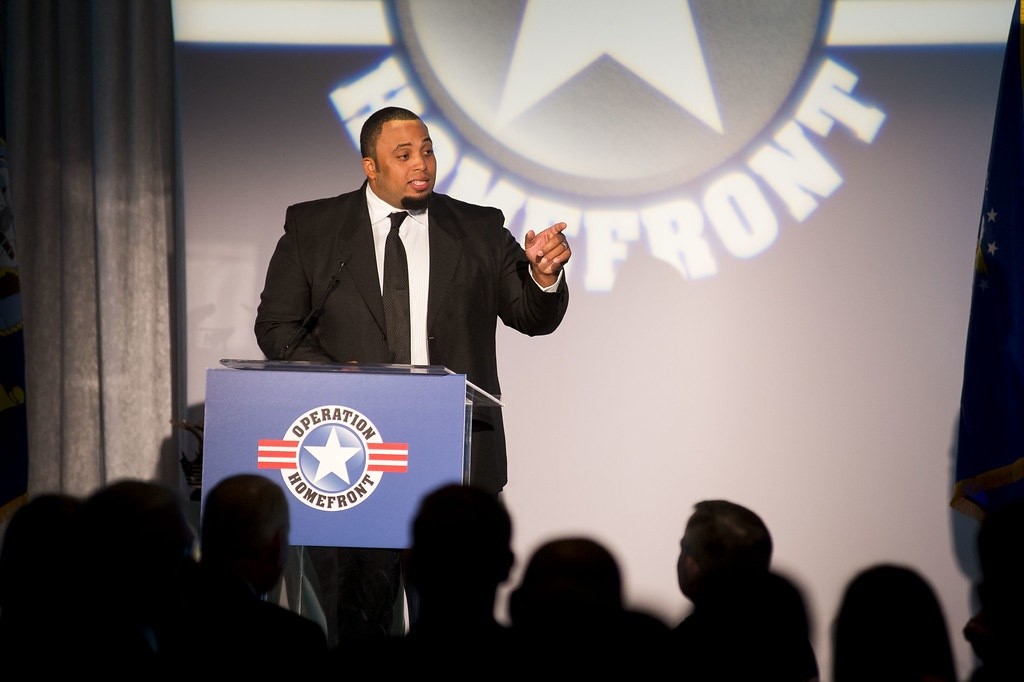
[382,211,412,365]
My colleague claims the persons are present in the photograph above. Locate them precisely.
[254,106,572,626]
[0,475,1024,682]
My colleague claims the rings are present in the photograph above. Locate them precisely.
[562,242,568,249]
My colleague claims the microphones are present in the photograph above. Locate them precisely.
[262,253,350,370]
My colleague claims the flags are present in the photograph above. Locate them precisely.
[950,0,1024,536]
